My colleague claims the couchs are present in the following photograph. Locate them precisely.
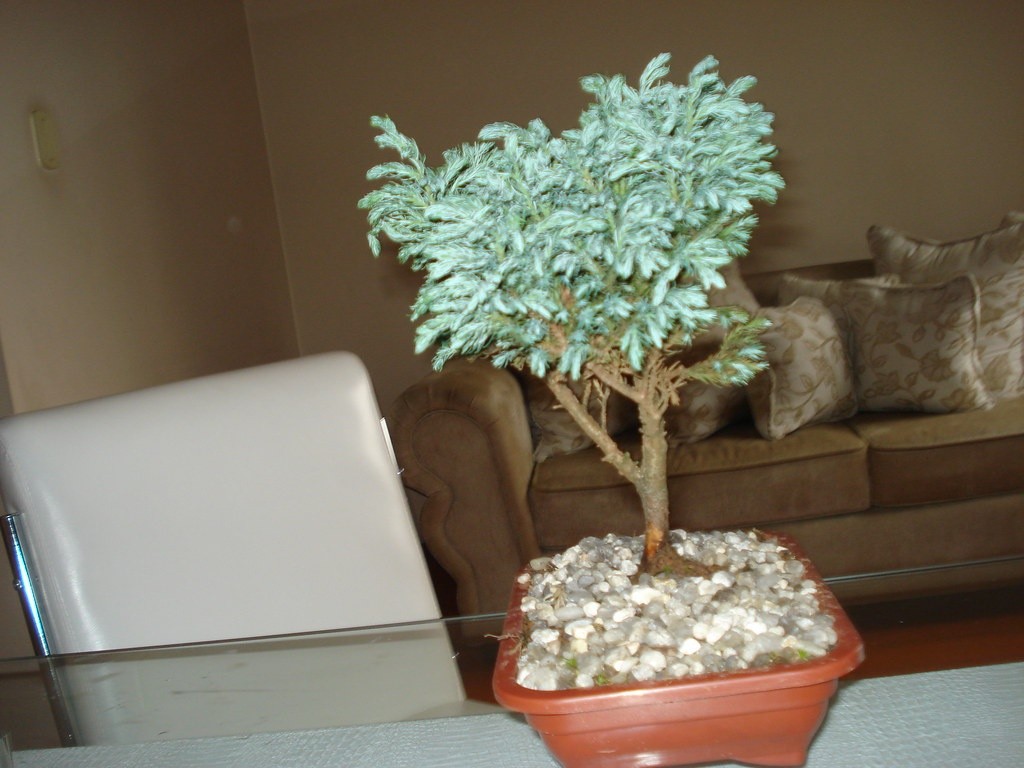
[386,210,1024,672]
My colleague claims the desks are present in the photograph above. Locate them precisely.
[0,556,1024,768]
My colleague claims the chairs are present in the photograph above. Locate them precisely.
[0,351,503,762]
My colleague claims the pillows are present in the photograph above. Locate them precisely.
[737,294,860,443]
[777,273,902,364]
[867,220,1024,402]
[643,256,761,446]
[477,340,643,465]
[837,269,997,414]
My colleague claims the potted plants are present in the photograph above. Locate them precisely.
[358,47,867,768]
